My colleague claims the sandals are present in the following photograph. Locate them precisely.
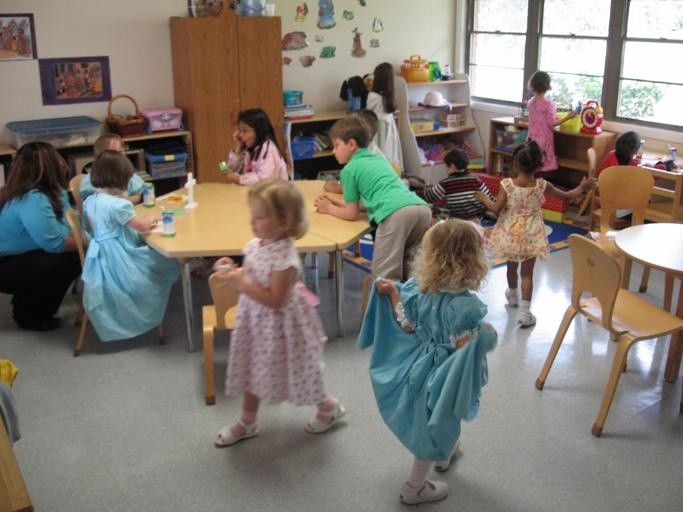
[215,418,261,447]
[304,397,347,434]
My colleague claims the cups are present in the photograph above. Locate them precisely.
[429,61,439,81]
[266,3,275,16]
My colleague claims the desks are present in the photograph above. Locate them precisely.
[131,180,380,353]
[614,222,682,381]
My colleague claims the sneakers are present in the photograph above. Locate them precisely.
[518,311,537,326]
[432,439,461,472]
[504,288,520,307]
[399,480,449,505]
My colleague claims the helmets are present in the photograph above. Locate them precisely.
[422,91,443,106]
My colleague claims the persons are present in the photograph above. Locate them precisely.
[412,149,498,223]
[356,218,497,505]
[599,130,674,222]
[79,133,145,205]
[524,71,577,172]
[474,137,597,327]
[315,113,432,285]
[210,175,347,447]
[0,141,90,331]
[366,62,405,172]
[352,107,386,161]
[227,108,289,186]
[81,149,228,343]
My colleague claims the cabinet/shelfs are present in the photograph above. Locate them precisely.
[280,109,343,181]
[490,115,616,228]
[392,71,488,185]
[116,129,196,205]
[0,144,19,191]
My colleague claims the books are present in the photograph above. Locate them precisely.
[144,149,191,178]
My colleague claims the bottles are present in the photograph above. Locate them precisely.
[442,64,451,80]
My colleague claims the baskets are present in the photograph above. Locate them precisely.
[104,94,146,138]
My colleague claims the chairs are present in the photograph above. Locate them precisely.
[64,206,168,355]
[67,172,84,212]
[200,268,238,407]
[598,165,653,260]
[631,162,682,294]
[533,232,682,437]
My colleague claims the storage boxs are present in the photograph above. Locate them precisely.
[5,114,104,147]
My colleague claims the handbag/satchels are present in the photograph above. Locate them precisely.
[400,55,429,83]
